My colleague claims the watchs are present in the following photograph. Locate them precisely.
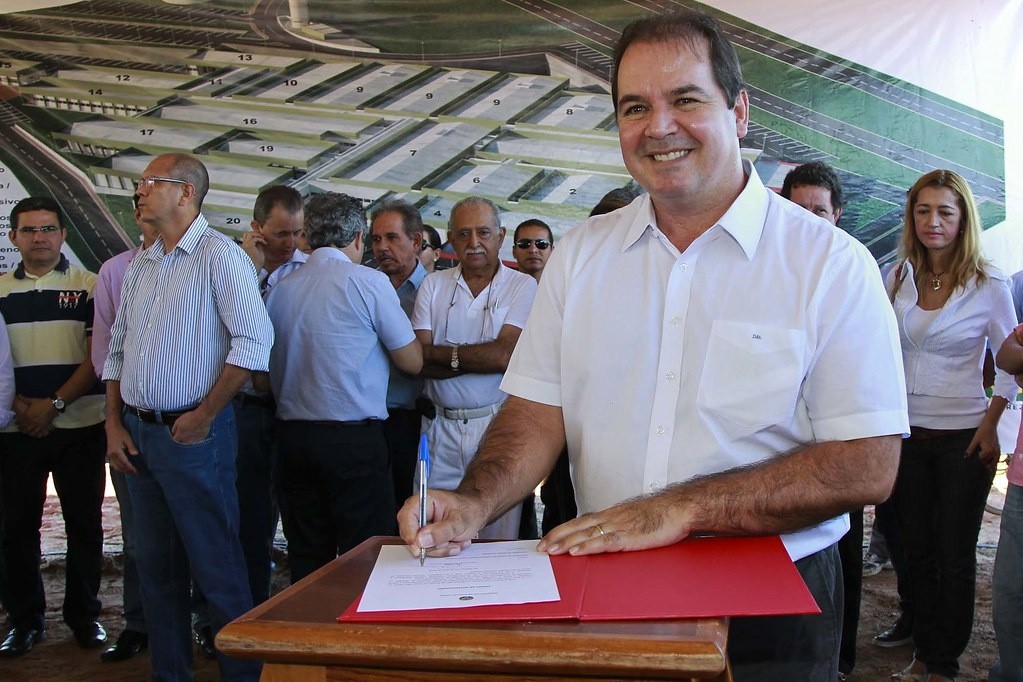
[450,348,460,376]
[49,393,66,413]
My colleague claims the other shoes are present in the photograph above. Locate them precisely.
[838,671,847,682]
[892,659,928,682]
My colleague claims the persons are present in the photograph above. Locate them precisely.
[503,188,638,541]
[782,161,863,682]
[266,190,423,587]
[91,194,214,662]
[101,153,276,682]
[410,197,537,539]
[983,270,1023,682]
[367,199,441,537]
[232,186,313,608]
[861,259,902,577]
[396,9,910,682]
[886,169,1019,682]
[0,312,16,631]
[0,197,107,658]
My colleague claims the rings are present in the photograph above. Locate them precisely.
[597,526,604,534]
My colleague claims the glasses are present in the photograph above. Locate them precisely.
[422,239,437,251]
[18,225,60,234]
[137,176,186,189]
[517,238,552,250]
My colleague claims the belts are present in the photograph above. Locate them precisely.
[123,405,199,423]
[235,393,272,405]
[438,401,501,420]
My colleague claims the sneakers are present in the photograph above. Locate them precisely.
[873,614,913,648]
[862,550,893,577]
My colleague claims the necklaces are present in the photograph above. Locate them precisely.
[925,260,944,291]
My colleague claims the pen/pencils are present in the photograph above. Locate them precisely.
[418,433,429,568]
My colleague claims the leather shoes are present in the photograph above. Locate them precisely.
[196,632,218,660]
[0,626,47,659]
[101,629,148,662]
[73,619,108,650]
